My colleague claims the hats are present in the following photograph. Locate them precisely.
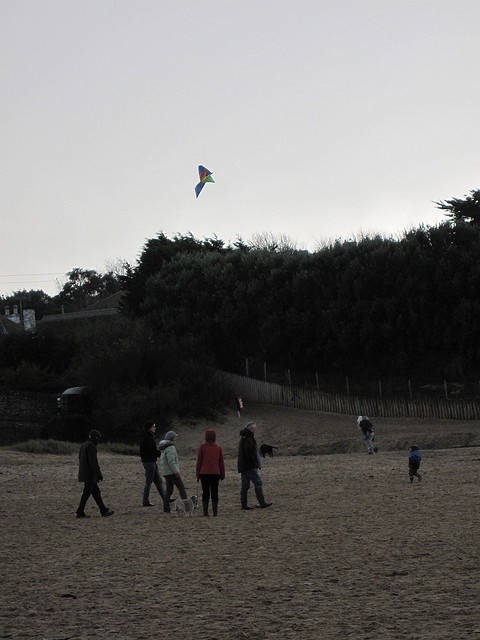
[246,422,256,430]
[411,446,419,452]
[357,416,362,423]
[164,431,178,441]
[143,420,155,431]
[88,430,102,445]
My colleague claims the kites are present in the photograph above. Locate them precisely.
[194,162,215,200]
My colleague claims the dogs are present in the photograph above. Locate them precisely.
[175,495,199,519]
[259,444,279,458]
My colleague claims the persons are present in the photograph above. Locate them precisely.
[75,429,115,518]
[404,440,424,484]
[139,420,176,507]
[354,413,378,455]
[236,421,273,511]
[195,429,226,517]
[157,429,188,513]
[232,392,247,417]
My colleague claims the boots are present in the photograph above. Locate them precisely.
[257,495,273,508]
[203,501,209,516]
[241,495,253,510]
[212,500,217,516]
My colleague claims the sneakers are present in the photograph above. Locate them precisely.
[143,501,156,506]
[76,515,90,518]
[169,499,176,502]
[100,509,114,517]
